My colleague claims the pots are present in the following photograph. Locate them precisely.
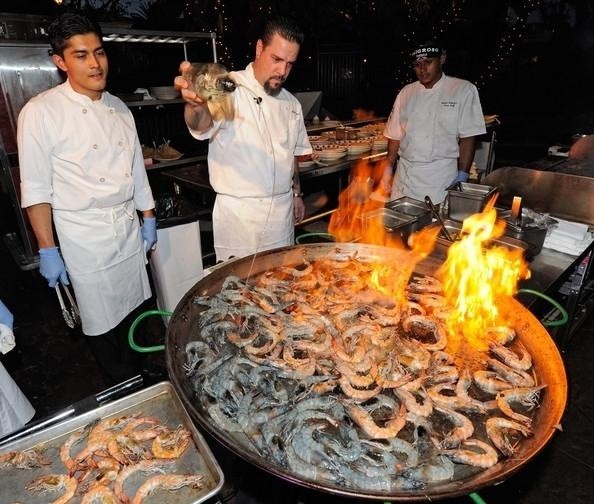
[129,232,568,504]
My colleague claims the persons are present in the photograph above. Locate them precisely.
[383,34,486,212]
[16,14,158,392]
[175,16,314,264]
[0,303,37,442]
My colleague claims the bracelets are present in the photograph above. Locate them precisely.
[293,193,304,198]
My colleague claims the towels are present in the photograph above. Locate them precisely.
[543,217,594,257]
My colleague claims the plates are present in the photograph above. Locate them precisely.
[151,86,180,101]
[485,119,495,124]
[154,154,182,163]
[298,121,388,167]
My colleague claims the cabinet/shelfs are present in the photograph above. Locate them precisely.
[95,29,217,171]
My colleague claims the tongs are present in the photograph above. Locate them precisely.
[55,276,82,328]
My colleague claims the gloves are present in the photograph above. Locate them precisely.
[379,165,393,193]
[448,170,468,186]
[38,245,68,288]
[140,216,158,252]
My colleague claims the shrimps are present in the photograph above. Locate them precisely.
[183,249,549,498]
[0,410,205,504]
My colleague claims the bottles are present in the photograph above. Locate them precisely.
[312,114,330,126]
[184,63,261,121]
